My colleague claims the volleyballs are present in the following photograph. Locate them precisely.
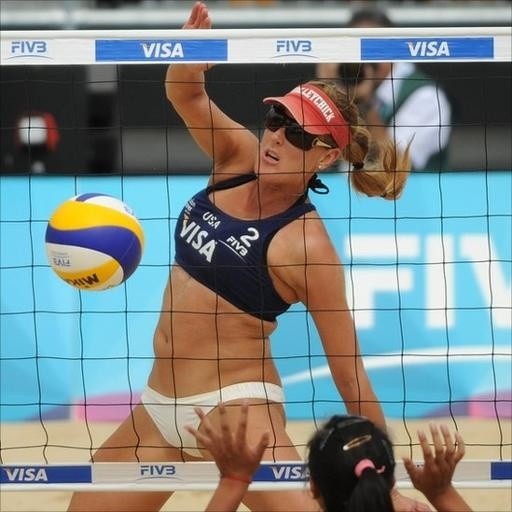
[43,190,146,293]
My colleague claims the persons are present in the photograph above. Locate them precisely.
[183,401,473,512]
[313,8,454,173]
[64,2,411,512]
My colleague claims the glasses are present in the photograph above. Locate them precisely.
[264,105,333,151]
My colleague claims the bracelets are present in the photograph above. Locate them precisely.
[219,474,252,484]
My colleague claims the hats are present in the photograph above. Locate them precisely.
[262,83,354,150]
